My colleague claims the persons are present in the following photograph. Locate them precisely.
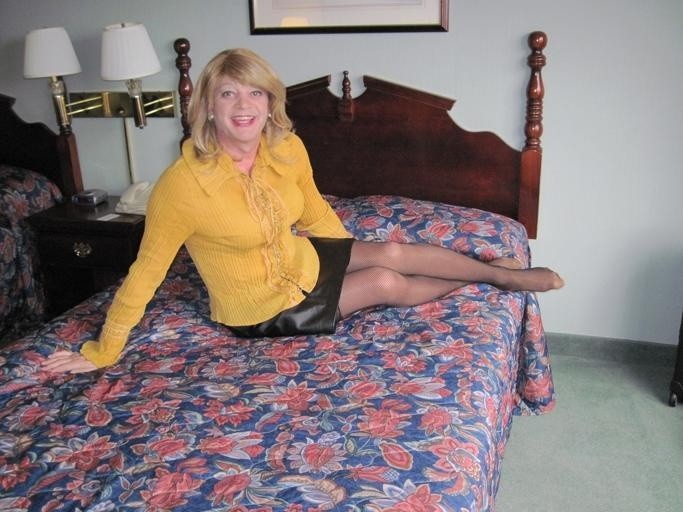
[38,47,566,374]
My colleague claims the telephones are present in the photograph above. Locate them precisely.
[115,181,157,216]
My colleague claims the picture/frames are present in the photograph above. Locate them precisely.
[247,0,450,36]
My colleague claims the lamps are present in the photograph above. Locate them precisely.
[23,21,178,130]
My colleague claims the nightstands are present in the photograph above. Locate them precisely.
[24,195,148,318]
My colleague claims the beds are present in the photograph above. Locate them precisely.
[1,31,547,511]
[1,93,84,349]
